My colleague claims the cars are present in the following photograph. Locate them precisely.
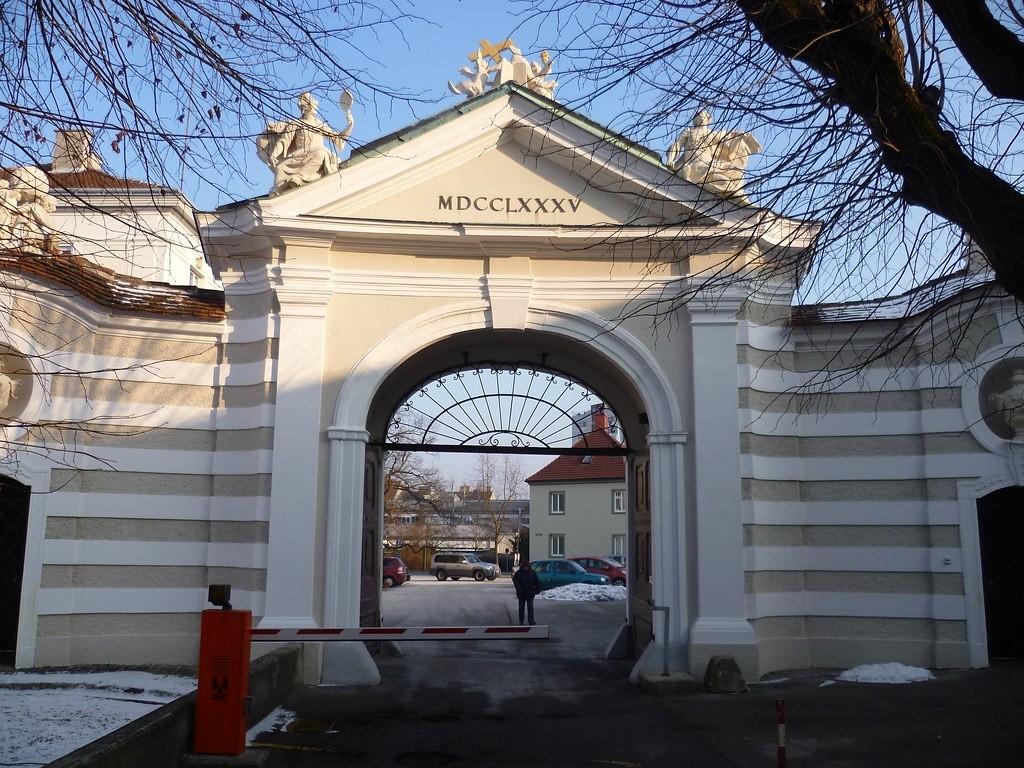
[566,556,626,588]
[511,566,520,577]
[383,557,411,588]
[530,560,610,589]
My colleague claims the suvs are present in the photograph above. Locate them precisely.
[430,552,501,581]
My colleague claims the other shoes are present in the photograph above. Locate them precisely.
[529,621,536,625]
[520,623,523,625]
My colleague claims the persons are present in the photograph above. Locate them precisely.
[512,559,541,625]
[268,93,352,196]
[540,557,602,574]
[446,45,558,101]
[665,109,741,191]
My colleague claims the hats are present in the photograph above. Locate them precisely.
[520,559,530,568]
